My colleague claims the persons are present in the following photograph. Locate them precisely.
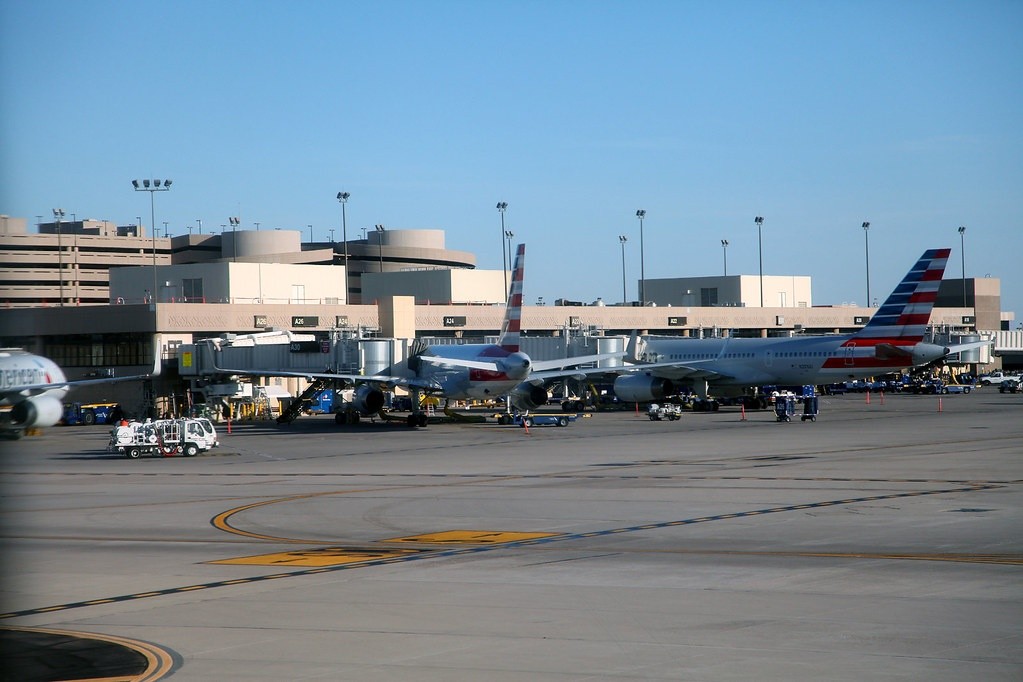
[971,377,977,388]
[787,389,796,416]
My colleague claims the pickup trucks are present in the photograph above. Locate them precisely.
[979,370,1022,386]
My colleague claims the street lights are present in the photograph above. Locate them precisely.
[163,221,169,237]
[635,209,646,307]
[130,178,173,304]
[187,227,193,234]
[196,219,201,234]
[336,191,351,303]
[958,226,966,308]
[155,227,161,238]
[504,230,515,271]
[53,207,65,306]
[308,224,312,243]
[70,212,75,222]
[720,239,728,276]
[495,201,509,302]
[361,228,366,240]
[329,229,335,242]
[254,223,260,231]
[101,219,108,236]
[136,217,141,238]
[358,235,361,240]
[229,216,240,261]
[755,215,764,307]
[618,235,628,306]
[327,236,330,242]
[220,224,226,233]
[861,222,870,308]
[375,224,385,273]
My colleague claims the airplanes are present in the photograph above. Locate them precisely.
[0,338,161,440]
[614,248,995,411]
[204,243,719,425]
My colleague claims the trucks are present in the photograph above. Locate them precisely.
[61,400,123,426]
[106,415,220,459]
